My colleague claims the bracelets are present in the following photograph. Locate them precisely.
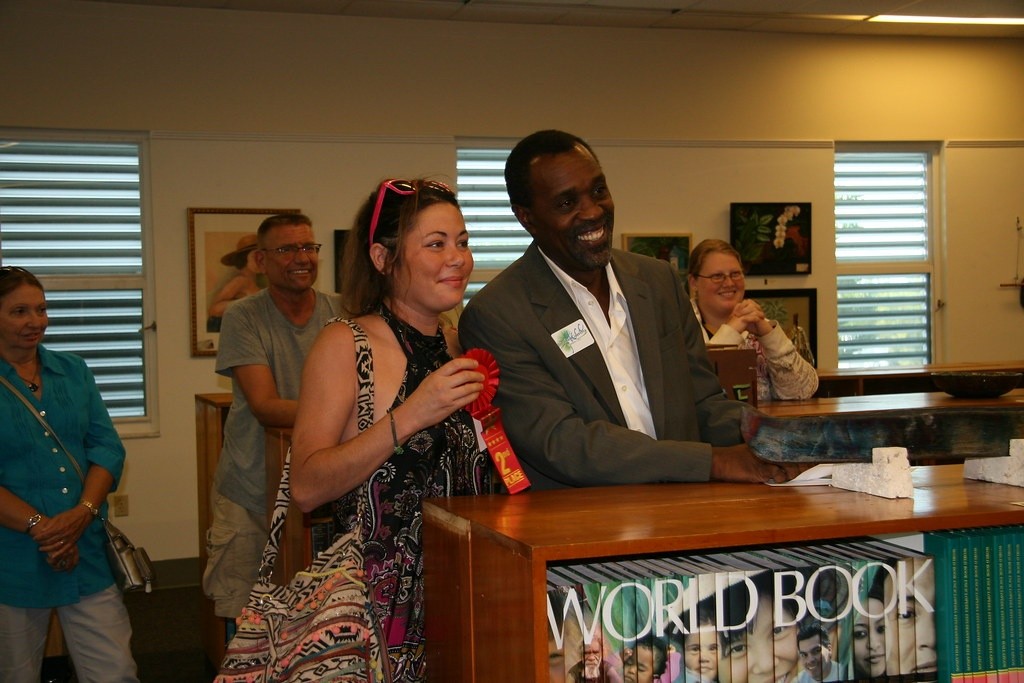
[390,409,404,454]
[25,512,41,535]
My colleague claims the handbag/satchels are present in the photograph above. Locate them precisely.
[214,532,391,683]
[104,523,153,593]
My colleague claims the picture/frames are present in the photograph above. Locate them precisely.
[730,201,812,276]
[742,287,817,369]
[186,206,300,356]
[620,232,693,280]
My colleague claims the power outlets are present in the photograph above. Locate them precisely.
[114,495,128,516]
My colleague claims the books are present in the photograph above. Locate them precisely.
[544,526,1024,683]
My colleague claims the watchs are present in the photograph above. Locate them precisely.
[79,498,99,517]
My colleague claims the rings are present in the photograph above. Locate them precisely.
[59,540,66,548]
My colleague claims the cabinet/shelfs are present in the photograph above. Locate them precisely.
[193,368,1024,683]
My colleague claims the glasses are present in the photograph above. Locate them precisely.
[698,270,741,284]
[262,243,322,255]
[369,180,451,246]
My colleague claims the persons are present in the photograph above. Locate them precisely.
[850,586,886,676]
[686,239,820,401]
[791,625,846,683]
[871,558,936,674]
[604,636,681,683]
[0,265,141,683]
[545,586,622,683]
[202,212,351,620]
[457,129,809,492]
[213,178,497,683]
[677,601,717,683]
[717,582,798,683]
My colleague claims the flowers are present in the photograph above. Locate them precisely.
[456,346,503,412]
